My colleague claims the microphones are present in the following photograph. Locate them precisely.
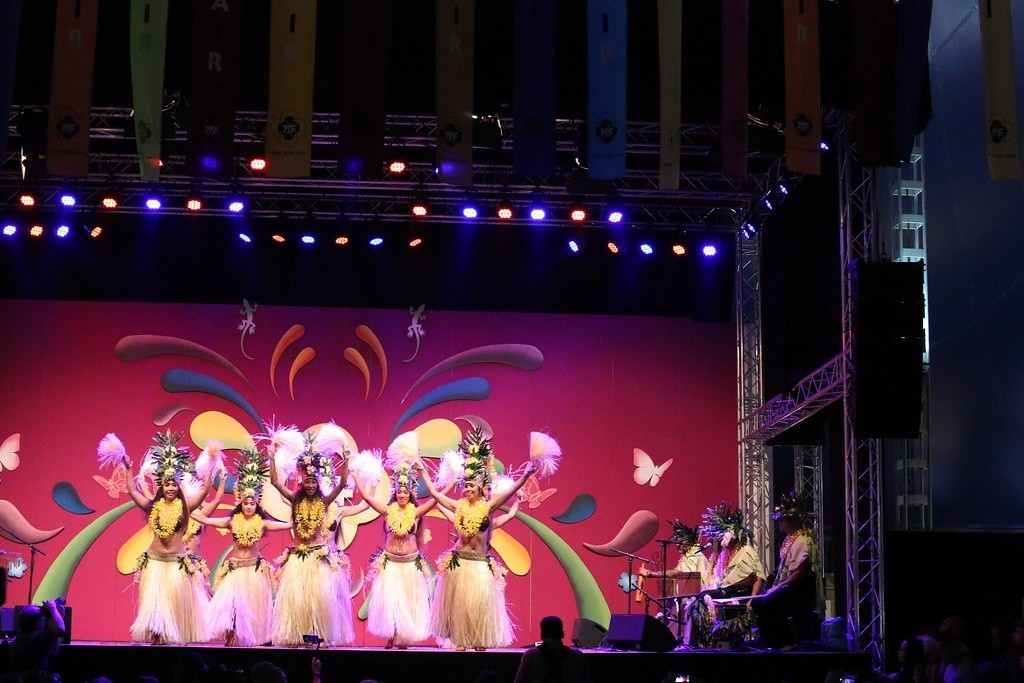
[716,583,726,596]
[694,543,712,554]
[667,617,686,625]
[655,553,659,572]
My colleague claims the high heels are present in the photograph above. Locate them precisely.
[225,632,236,647]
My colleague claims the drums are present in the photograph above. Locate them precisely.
[676,570,702,596]
[713,603,749,641]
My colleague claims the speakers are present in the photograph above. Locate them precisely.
[571,617,609,648]
[13,605,71,644]
[607,614,678,652]
[853,257,924,438]
[0,607,15,638]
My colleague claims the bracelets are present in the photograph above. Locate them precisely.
[647,569,652,578]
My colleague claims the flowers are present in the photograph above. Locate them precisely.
[130,423,825,650]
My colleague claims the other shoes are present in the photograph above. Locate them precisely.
[385,638,395,648]
[151,634,161,645]
[475,645,486,651]
[292,645,298,648]
[779,644,797,652]
[306,644,312,648]
[456,645,466,651]
[398,643,407,649]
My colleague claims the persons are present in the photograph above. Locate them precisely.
[514,615,590,683]
[413,461,535,652]
[637,520,709,593]
[674,501,769,652]
[0,601,1024,683]
[436,496,524,583]
[188,445,294,647]
[121,455,213,646]
[750,492,823,652]
[268,437,357,647]
[349,468,461,650]
[279,482,378,587]
[138,470,229,602]
